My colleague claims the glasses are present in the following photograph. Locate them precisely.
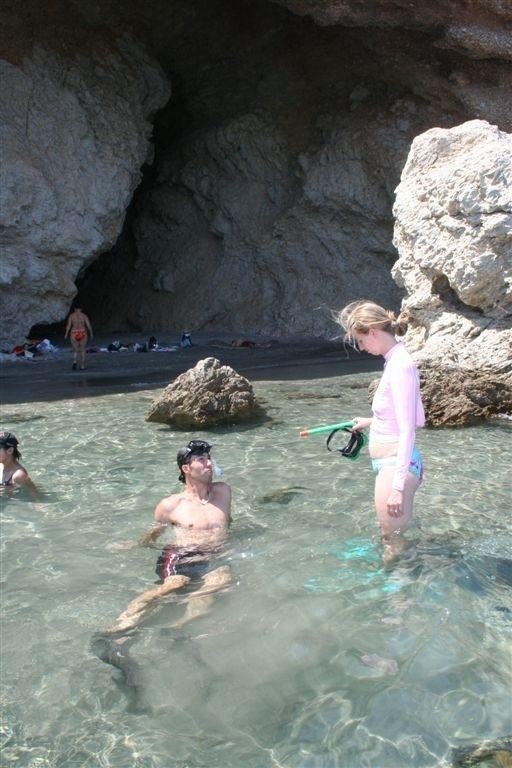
[326,427,367,461]
[183,440,212,459]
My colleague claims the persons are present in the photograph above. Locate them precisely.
[64,302,93,369]
[180,329,192,348]
[107,441,231,658]
[0,432,40,501]
[142,336,156,352]
[338,299,424,626]
